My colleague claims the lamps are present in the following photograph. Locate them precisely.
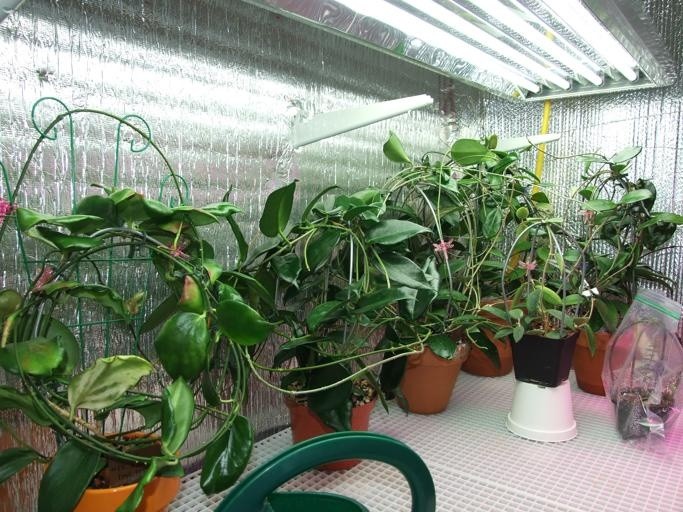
[240,0,676,104]
